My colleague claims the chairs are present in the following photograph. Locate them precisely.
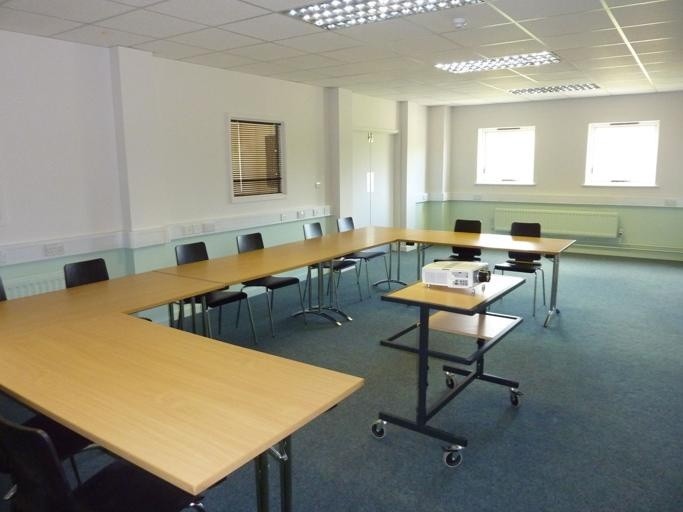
[0,219,587,512]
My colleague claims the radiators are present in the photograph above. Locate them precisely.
[493,205,622,240]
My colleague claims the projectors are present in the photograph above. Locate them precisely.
[422,261,490,289]
